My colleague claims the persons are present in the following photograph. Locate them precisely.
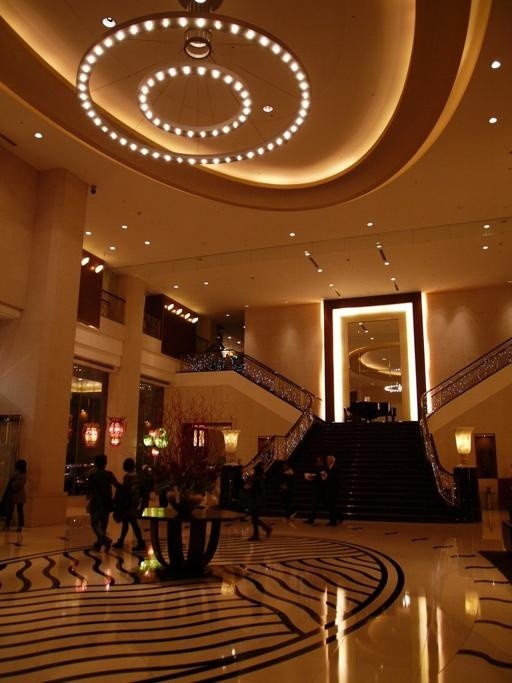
[4,458,27,531]
[303,455,341,526]
[84,454,120,553]
[246,463,272,541]
[112,458,146,550]
[142,455,181,507]
[281,462,300,520]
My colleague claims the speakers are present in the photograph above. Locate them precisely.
[219,465,243,509]
[454,467,482,522]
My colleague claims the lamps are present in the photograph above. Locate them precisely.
[144,419,170,457]
[452,426,474,466]
[82,420,100,449]
[222,428,241,462]
[107,414,127,446]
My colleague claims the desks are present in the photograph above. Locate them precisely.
[143,507,248,581]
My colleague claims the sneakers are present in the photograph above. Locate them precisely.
[92,536,148,552]
[288,512,344,527]
[247,527,273,541]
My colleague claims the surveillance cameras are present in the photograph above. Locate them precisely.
[91,186,96,194]
